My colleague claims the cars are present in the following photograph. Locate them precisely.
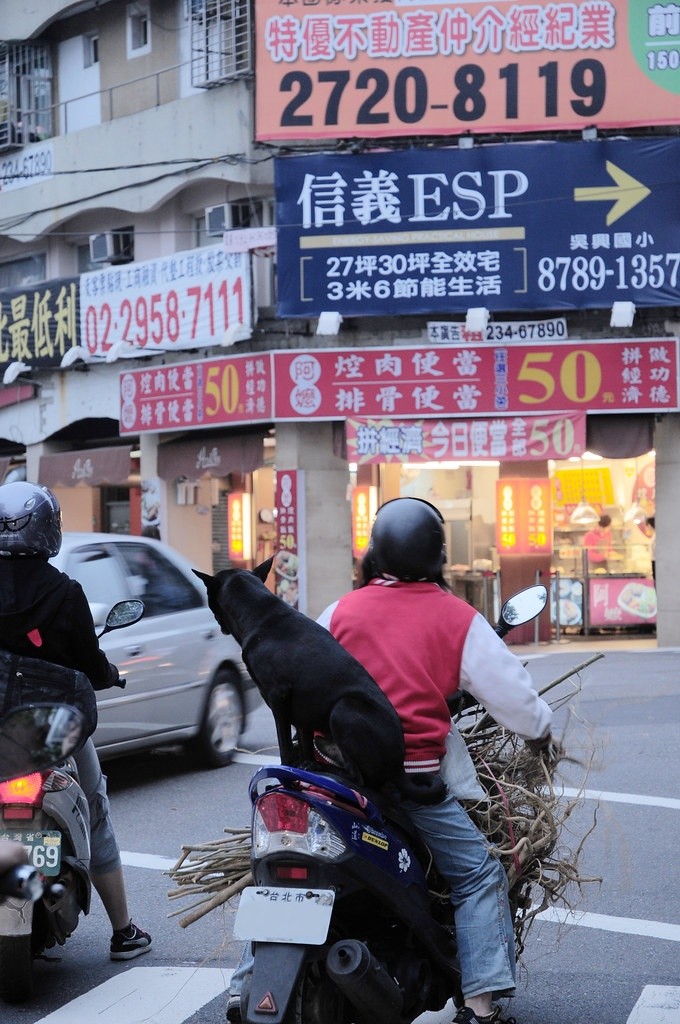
[40,532,262,771]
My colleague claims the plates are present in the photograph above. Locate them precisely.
[276,579,298,607]
[553,579,572,598]
[260,508,275,524]
[551,599,581,625]
[275,549,298,581]
[571,582,583,596]
[617,581,657,618]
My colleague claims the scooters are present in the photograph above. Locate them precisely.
[220,583,551,1024]
[0,599,149,1009]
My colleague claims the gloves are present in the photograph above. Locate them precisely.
[524,730,566,764]
[93,663,118,690]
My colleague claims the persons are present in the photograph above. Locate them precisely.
[583,514,614,572]
[646,517,657,634]
[0,481,152,960]
[225,497,553,1024]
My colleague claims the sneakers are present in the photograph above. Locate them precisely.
[109,918,153,959]
[450,1003,516,1024]
[226,996,241,1022]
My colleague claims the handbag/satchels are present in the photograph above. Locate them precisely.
[0,650,98,737]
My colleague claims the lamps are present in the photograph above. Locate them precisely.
[315,311,349,335]
[609,301,639,328]
[464,307,494,332]
[2,342,190,386]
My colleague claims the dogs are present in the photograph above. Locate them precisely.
[190,555,449,807]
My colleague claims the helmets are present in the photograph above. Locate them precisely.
[0,481,63,556]
[371,497,444,582]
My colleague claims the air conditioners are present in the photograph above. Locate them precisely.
[0,119,17,147]
[205,202,240,236]
[88,229,122,261]
